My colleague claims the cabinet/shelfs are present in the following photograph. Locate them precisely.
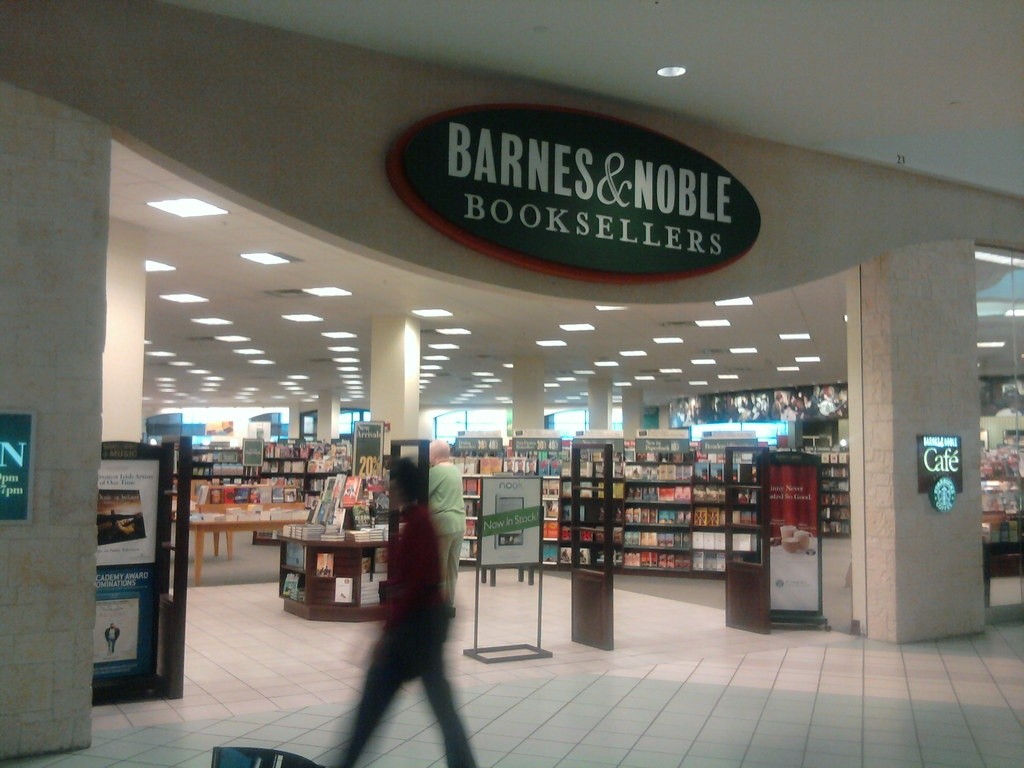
[143,408,853,623]
[977,446,1024,580]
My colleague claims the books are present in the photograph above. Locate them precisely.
[171,429,851,573]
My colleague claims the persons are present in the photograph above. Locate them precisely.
[420,439,468,615]
[325,457,472,768]
